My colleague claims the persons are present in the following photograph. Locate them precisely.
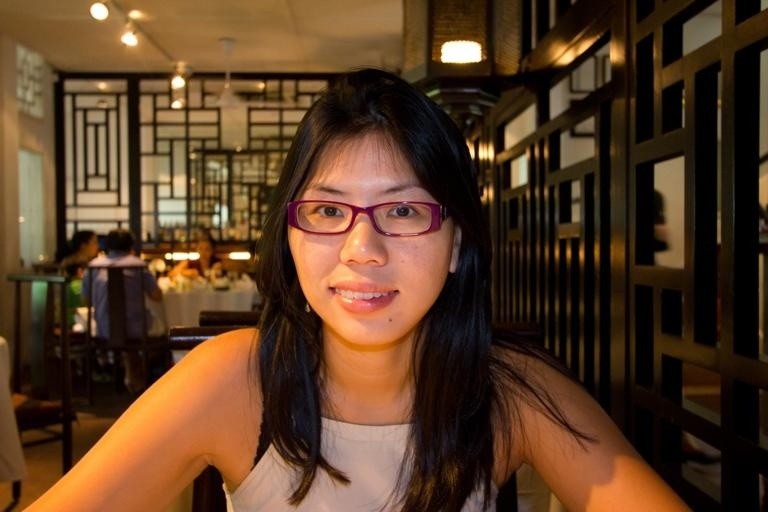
[81,230,166,391]
[62,252,105,378]
[168,232,225,279]
[51,228,98,332]
[20,69,692,510]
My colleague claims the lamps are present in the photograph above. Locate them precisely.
[90,0,189,88]
[215,37,240,106]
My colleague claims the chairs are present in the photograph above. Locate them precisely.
[6,257,518,512]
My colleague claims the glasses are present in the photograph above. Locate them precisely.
[287,200,449,237]
